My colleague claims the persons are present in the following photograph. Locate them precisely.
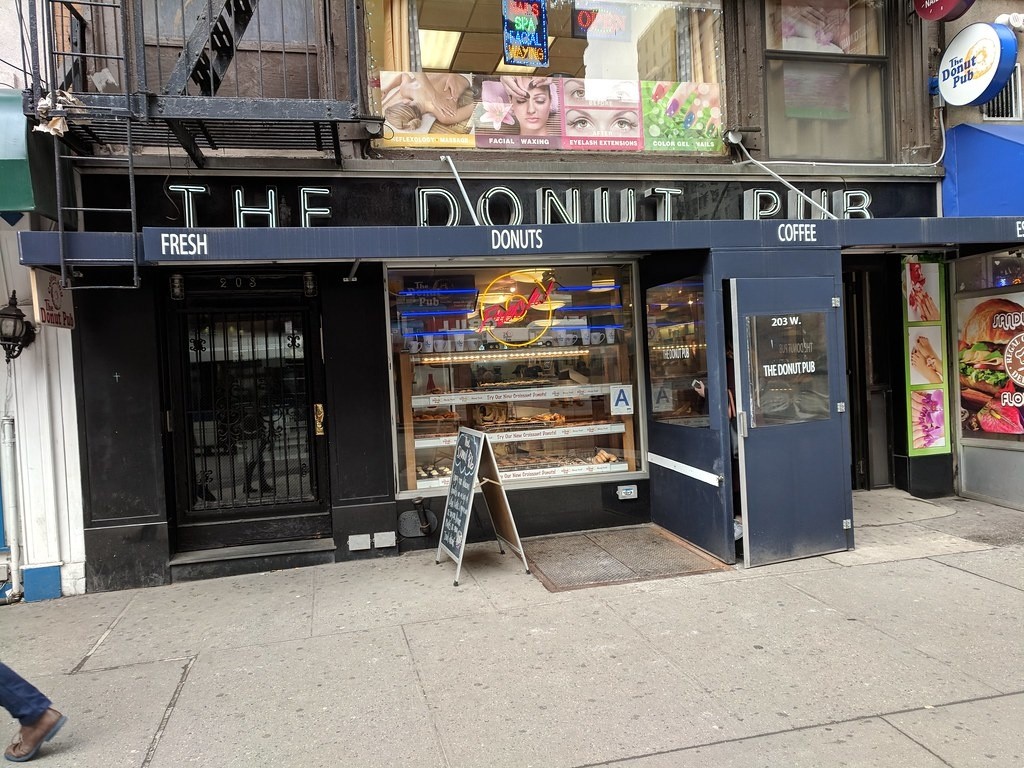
[477,361,591,383]
[0,663,66,762]
[786,1,827,30]
[695,338,763,541]
[910,288,944,448]
[380,71,720,139]
[242,413,275,493]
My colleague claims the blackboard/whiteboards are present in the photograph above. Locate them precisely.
[432,426,485,565]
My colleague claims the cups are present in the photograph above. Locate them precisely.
[409,341,421,353]
[445,340,451,351]
[557,330,566,346]
[590,333,605,344]
[605,328,615,344]
[468,338,483,351]
[566,334,578,345]
[581,329,590,345]
[454,334,464,351]
[433,340,447,352]
[423,335,433,353]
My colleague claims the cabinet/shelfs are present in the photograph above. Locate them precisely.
[400,343,636,490]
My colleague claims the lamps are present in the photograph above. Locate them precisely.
[170,274,185,301]
[0,290,37,365]
[304,272,317,296]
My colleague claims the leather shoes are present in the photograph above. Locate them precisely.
[4,707,68,762]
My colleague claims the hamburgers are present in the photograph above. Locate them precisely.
[961,300,1024,404]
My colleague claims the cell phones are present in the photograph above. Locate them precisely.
[692,380,701,389]
